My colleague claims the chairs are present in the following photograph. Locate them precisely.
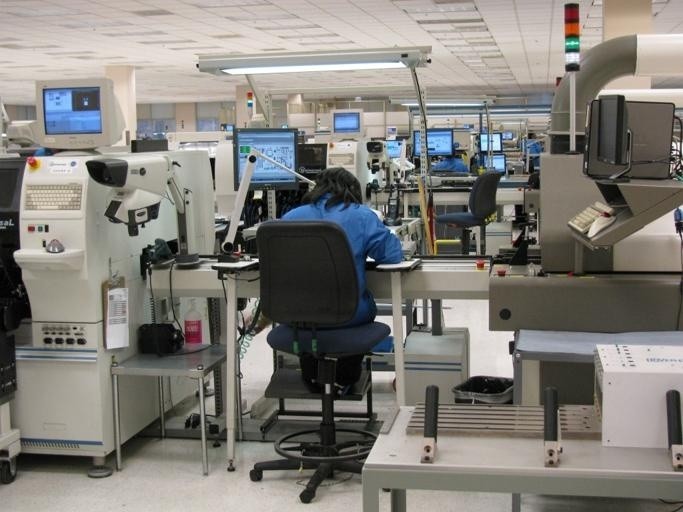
[250,221,391,503]
[435,169,506,254]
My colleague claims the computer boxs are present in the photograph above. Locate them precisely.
[582,100,675,180]
[551,135,587,154]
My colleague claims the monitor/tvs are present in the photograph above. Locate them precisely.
[7,120,36,145]
[331,109,366,140]
[385,124,513,175]
[298,143,328,181]
[596,94,633,183]
[0,160,27,213]
[234,128,299,192]
[35,78,126,149]
[226,124,234,132]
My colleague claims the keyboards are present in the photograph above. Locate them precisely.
[24,185,83,211]
[567,201,615,235]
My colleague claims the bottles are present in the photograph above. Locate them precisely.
[184,300,202,350]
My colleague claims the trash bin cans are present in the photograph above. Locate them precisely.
[451,376,513,402]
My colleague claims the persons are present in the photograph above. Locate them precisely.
[431,148,470,172]
[517,132,544,168]
[280,166,404,398]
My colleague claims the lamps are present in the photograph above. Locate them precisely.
[196,46,435,78]
[387,93,553,120]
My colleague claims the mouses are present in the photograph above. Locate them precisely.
[586,212,616,239]
[46,239,65,253]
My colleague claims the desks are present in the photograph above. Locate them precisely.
[110,344,242,475]
